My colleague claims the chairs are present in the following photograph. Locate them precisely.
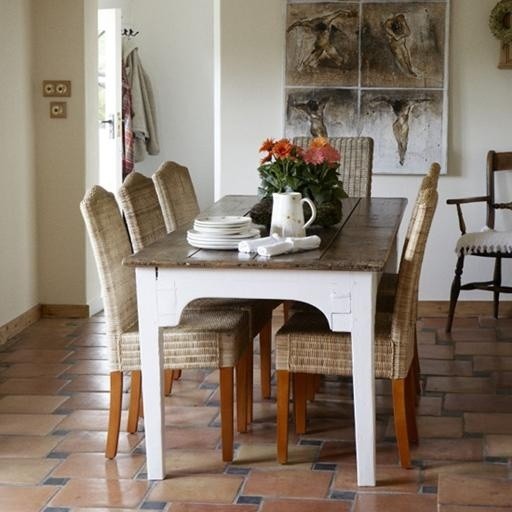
[152,160,200,234]
[80,185,248,463]
[118,172,272,427]
[275,188,438,468]
[284,163,441,406]
[446,144,512,335]
[292,135,373,199]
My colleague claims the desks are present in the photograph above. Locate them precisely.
[122,193,412,486]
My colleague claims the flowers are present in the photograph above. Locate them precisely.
[257,138,349,204]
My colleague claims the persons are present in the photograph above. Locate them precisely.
[384,14,418,77]
[369,98,433,166]
[295,12,354,73]
[295,97,330,137]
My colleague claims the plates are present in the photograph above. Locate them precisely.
[186,215,262,250]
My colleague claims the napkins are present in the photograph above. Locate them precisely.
[286,235,321,252]
[238,235,276,252]
[258,241,293,256]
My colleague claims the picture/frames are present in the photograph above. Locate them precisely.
[282,0,450,176]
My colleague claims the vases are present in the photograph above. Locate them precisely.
[251,197,342,227]
